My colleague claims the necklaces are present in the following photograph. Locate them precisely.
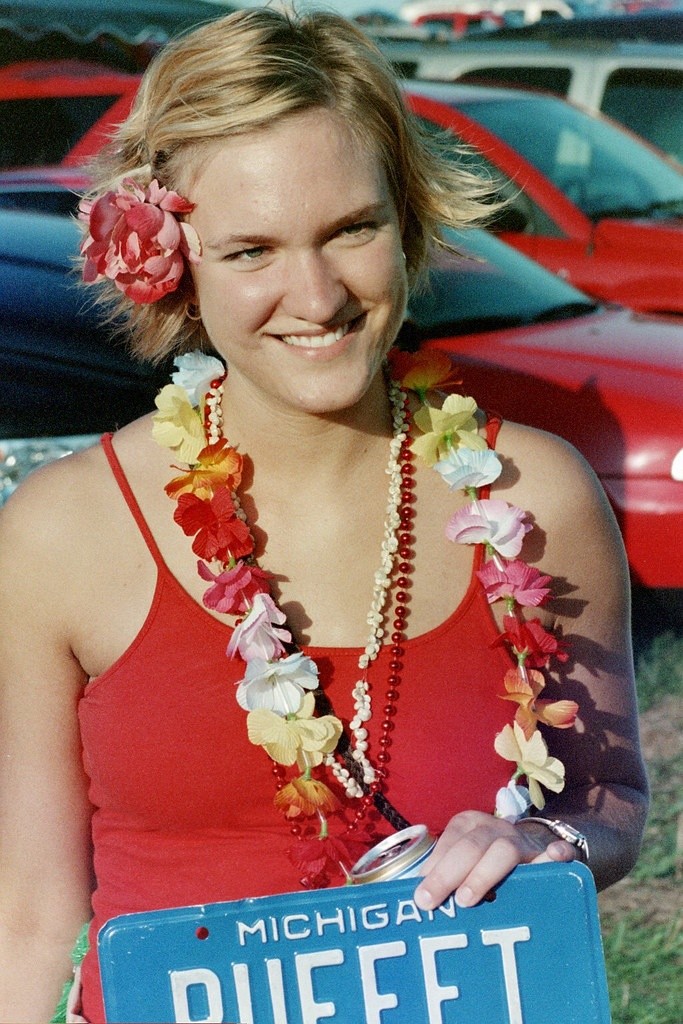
[147,346,580,888]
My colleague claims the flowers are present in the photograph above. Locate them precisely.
[76,179,203,306]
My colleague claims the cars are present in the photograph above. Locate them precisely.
[1,168,682,598]
[0,1,681,316]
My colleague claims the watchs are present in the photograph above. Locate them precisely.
[515,817,590,862]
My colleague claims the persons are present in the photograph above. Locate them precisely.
[1,8,650,1024]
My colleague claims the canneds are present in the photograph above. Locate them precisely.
[351,823,437,885]
